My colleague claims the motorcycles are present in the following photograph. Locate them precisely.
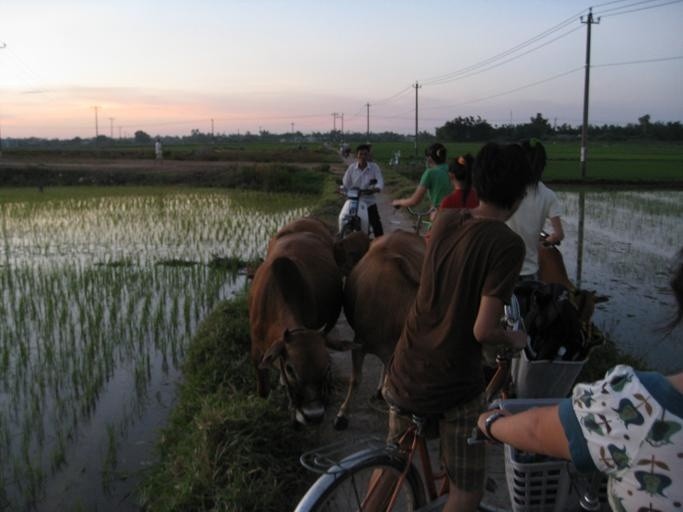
[334,179,379,240]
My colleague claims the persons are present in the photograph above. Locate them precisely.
[475,247,683,511]
[336,141,384,242]
[504,138,565,329]
[390,141,455,230]
[355,141,532,512]
[423,152,479,246]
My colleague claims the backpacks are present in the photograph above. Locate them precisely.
[512,280,598,363]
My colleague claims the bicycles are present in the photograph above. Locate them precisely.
[395,205,436,236]
[467,397,613,512]
[294,276,606,512]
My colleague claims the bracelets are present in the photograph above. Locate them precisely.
[484,411,505,445]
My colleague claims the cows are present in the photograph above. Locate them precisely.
[247,217,428,430]
[538,237,611,323]
